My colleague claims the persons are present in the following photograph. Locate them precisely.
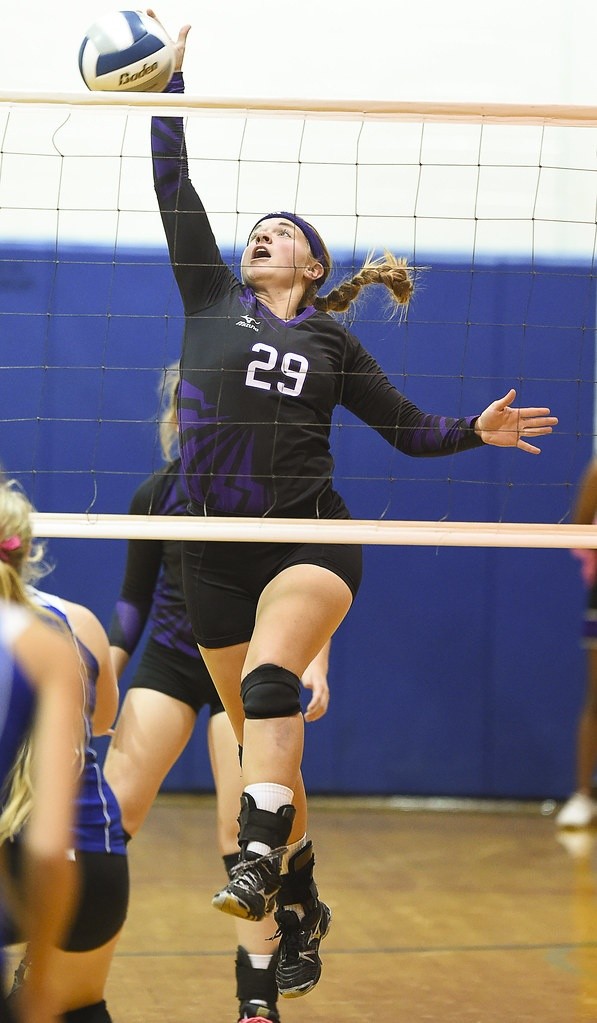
[0,479,90,1023]
[19,579,133,1023]
[0,6,564,1023]
[547,442,597,829]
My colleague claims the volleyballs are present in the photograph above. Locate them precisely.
[78,11,176,93]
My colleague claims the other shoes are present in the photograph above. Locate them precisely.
[554,831,597,858]
[554,789,597,828]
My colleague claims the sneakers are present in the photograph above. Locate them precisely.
[235,1001,280,1023]
[266,901,331,1000]
[209,843,279,924]
